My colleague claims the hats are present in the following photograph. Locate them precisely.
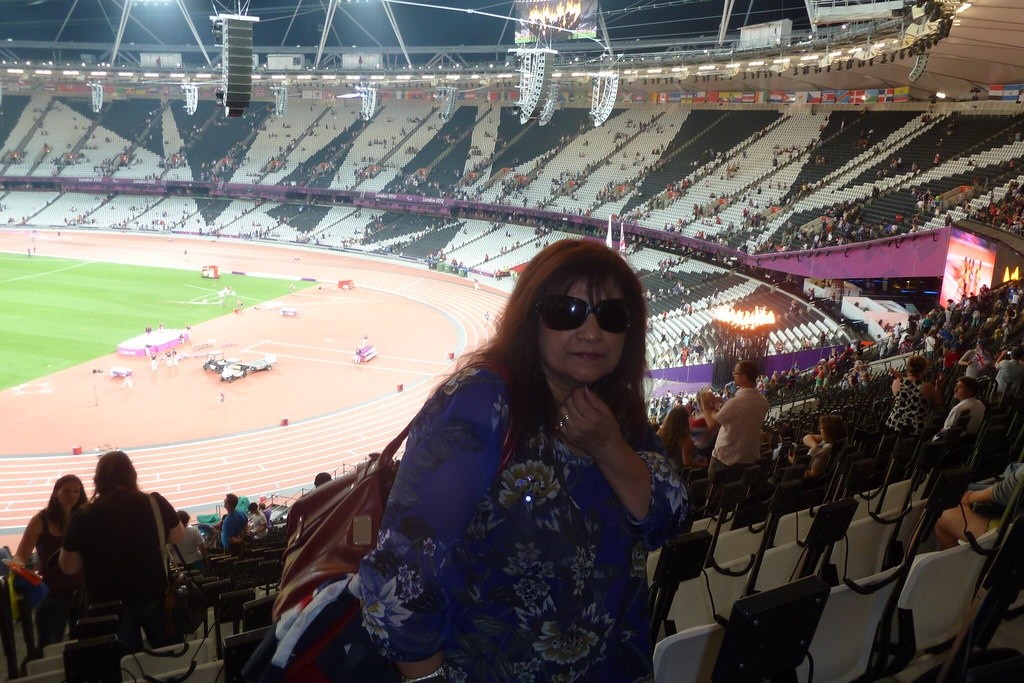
[820,358,826,362]
[854,360,862,365]
[977,338,987,346]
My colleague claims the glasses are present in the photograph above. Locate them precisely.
[732,371,744,376]
[818,426,824,430]
[535,294,634,333]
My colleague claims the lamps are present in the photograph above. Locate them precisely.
[892,9,904,17]
[905,0,953,57]
[620,50,904,85]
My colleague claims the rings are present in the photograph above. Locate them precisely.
[561,414,570,422]
[556,420,565,430]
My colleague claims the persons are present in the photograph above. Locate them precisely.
[30,101,1024,572]
[58,450,186,653]
[362,237,695,683]
[13,474,92,679]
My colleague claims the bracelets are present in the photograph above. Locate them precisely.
[400,663,450,683]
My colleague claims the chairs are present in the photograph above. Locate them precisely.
[0,453,1024,683]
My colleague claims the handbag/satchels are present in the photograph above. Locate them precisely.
[968,483,1000,512]
[167,542,208,634]
[160,576,178,642]
[271,359,520,627]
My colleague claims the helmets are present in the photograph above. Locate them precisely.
[818,365,825,372]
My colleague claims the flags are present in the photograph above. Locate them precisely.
[2,75,1024,105]
